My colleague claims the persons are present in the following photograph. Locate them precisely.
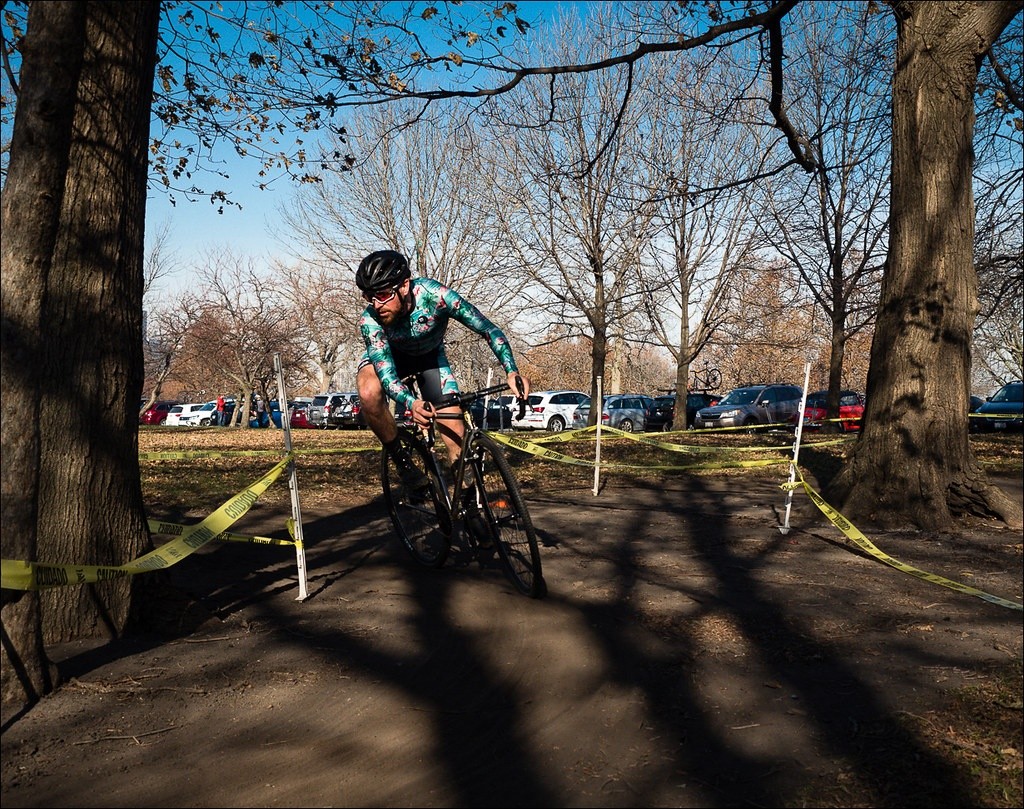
[358,250,529,510]
[216,394,224,426]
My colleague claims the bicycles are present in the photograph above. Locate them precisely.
[688,360,722,390]
[380,374,542,598]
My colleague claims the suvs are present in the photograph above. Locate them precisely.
[693,382,804,434]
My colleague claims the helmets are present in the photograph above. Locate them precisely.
[356,250,411,291]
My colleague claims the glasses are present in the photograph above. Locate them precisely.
[362,281,404,306]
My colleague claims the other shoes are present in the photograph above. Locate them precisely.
[464,512,490,536]
[396,453,429,490]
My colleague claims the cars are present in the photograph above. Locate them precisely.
[643,392,724,433]
[139,398,149,409]
[164,392,371,430]
[966,396,995,434]
[140,400,187,426]
[467,395,515,429]
[972,380,1024,432]
[571,394,654,433]
[510,390,591,432]
[786,389,864,435]
